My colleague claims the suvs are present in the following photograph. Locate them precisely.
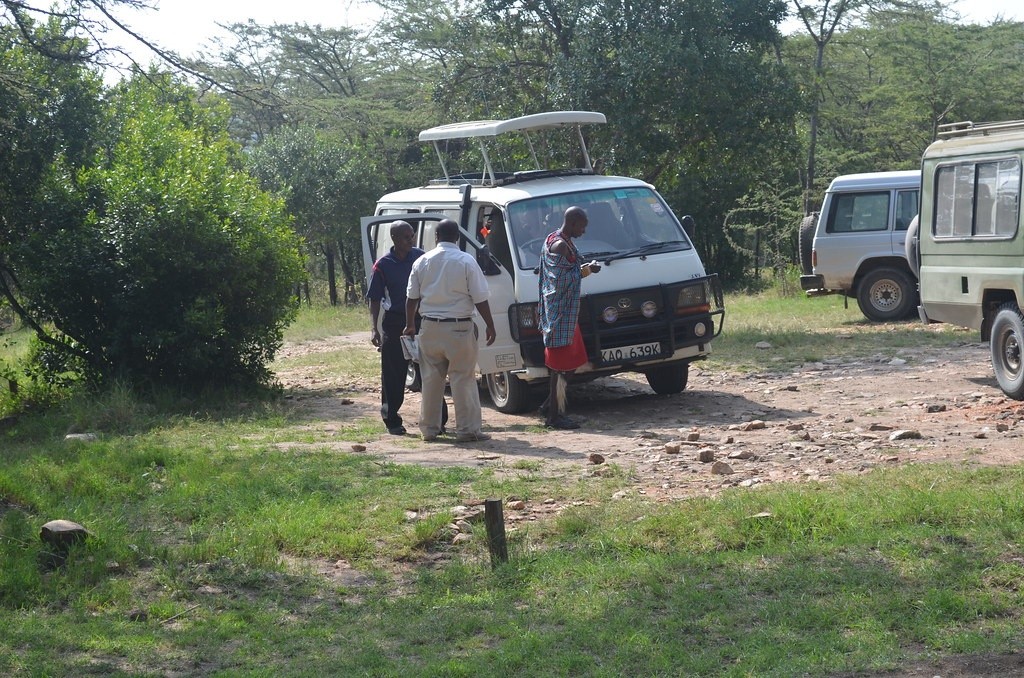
[796,169,922,322]
[904,117,1024,400]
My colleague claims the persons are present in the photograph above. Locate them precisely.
[365,221,448,435]
[402,220,496,440]
[537,205,600,428]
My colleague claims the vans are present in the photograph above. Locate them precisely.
[359,183,726,416]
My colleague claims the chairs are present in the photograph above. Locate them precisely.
[582,201,629,250]
[895,217,912,230]
[484,210,532,282]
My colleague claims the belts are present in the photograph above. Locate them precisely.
[420,316,471,322]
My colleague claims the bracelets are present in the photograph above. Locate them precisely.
[581,266,592,278]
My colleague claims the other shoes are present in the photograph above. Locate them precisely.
[457,433,491,442]
[421,433,436,441]
[389,426,406,435]
[537,406,581,429]
